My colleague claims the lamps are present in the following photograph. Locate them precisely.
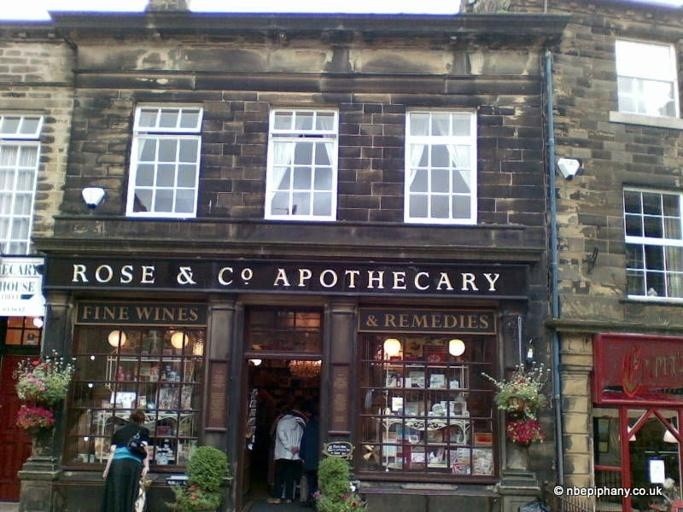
[107,329,127,347]
[662,410,679,444]
[557,156,580,181]
[618,424,637,441]
[382,334,401,355]
[167,325,188,350]
[287,359,321,381]
[79,184,106,211]
[447,335,465,357]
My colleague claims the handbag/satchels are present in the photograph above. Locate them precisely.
[127,438,147,458]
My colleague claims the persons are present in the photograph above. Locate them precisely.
[98,409,151,511]
[298,411,320,507]
[265,407,305,504]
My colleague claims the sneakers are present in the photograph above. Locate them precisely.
[285,499,292,503]
[266,497,280,504]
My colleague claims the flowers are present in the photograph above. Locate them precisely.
[10,348,77,433]
[481,354,554,447]
